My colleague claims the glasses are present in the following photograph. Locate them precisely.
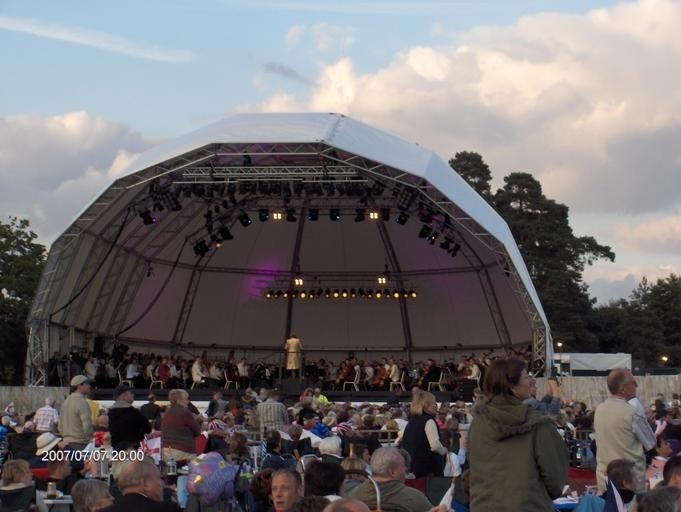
[524,375,531,382]
[431,404,436,409]
[620,379,637,387]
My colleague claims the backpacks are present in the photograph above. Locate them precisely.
[441,450,461,478]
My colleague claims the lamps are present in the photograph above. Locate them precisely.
[264,269,417,300]
[136,177,461,258]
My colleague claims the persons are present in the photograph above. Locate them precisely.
[1,332,681,510]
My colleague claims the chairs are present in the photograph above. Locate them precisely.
[343,371,360,391]
[223,369,232,390]
[190,382,199,390]
[389,371,406,392]
[149,369,166,389]
[117,369,134,388]
[477,370,482,393]
[428,371,447,392]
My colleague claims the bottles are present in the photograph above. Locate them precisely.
[166,456,177,475]
[46,482,57,500]
[84,449,108,477]
[576,448,582,468]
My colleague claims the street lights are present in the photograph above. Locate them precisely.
[662,356,669,368]
[556,341,564,381]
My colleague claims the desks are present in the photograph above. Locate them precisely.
[553,495,579,512]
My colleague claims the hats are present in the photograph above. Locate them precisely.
[288,424,302,439]
[70,374,87,386]
[34,432,62,457]
[111,383,133,398]
[336,422,351,437]
